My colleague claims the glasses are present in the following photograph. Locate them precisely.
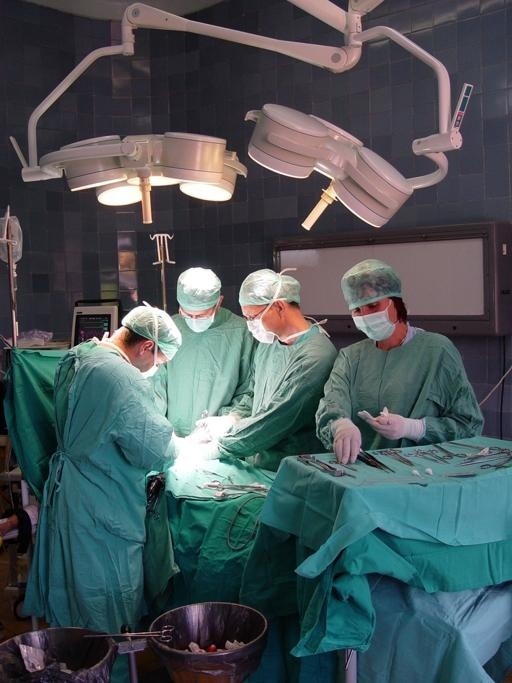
[240,308,268,322]
[181,307,216,320]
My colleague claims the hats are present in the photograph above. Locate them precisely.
[238,268,302,307]
[120,304,184,362]
[176,266,222,312]
[339,258,403,312]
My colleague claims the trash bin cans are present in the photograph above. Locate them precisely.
[1,624,118,682]
[146,600,272,683]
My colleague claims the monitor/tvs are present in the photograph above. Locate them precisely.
[71,305,118,348]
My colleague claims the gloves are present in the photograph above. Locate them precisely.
[329,415,363,465]
[356,405,426,444]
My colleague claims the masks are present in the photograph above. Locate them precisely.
[182,311,217,334]
[246,304,275,346]
[134,342,160,378]
[351,299,400,343]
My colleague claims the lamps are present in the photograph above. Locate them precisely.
[8,0,473,231]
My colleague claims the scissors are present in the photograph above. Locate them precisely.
[330,457,359,472]
[206,479,266,491]
[368,440,512,472]
[82,624,176,643]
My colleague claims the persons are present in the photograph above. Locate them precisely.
[199,269,340,470]
[316,259,486,463]
[24,307,183,683]
[145,265,255,432]
